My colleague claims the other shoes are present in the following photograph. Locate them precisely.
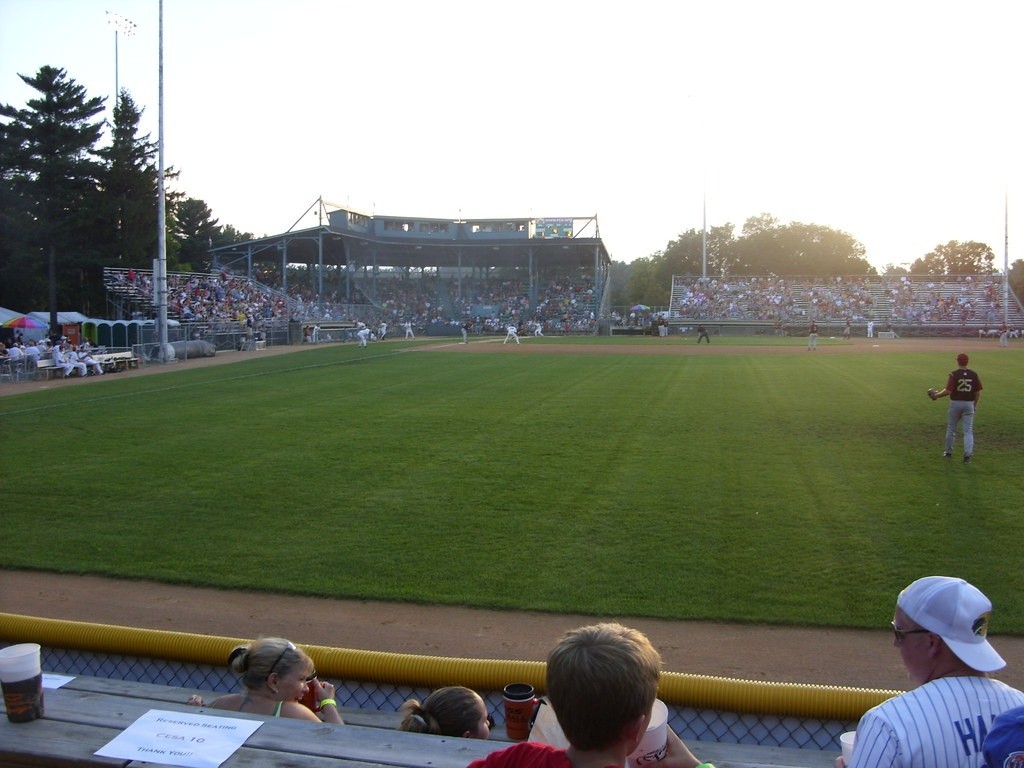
[963,457,970,464]
[944,453,951,459]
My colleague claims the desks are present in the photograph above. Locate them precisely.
[0,673,840,768]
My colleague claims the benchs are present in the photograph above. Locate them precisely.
[670,282,1024,325]
[0,349,139,382]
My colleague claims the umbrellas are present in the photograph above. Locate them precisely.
[2,316,49,340]
[629,304,651,311]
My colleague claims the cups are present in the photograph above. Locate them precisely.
[299,670,322,715]
[839,730,857,768]
[626,697,669,768]
[0,642,45,723]
[503,682,540,740]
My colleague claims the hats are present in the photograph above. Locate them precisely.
[956,353,969,362]
[897,576,1007,671]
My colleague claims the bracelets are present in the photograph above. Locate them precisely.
[696,763,716,768]
[319,698,337,711]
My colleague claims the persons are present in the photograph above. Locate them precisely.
[808,319,818,351]
[927,353,983,464]
[835,576,1024,768]
[398,685,491,740]
[189,637,345,724]
[998,322,1008,347]
[117,263,669,348]
[466,622,716,768]
[674,276,1024,338]
[696,324,711,343]
[0,330,107,378]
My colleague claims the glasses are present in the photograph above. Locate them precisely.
[890,621,930,639]
[266,641,297,682]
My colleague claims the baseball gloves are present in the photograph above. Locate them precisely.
[927,387,939,400]
